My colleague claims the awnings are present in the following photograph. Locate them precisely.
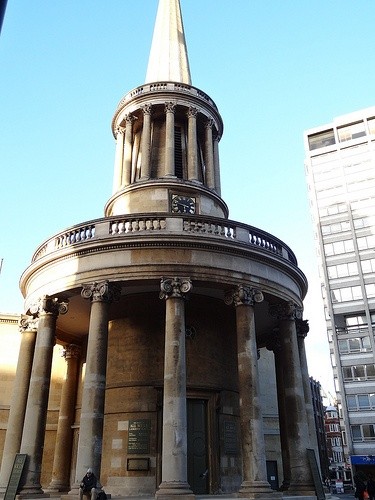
[351,455,375,464]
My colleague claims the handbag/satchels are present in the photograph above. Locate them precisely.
[96,492,108,500]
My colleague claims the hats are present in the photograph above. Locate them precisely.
[86,469,93,473]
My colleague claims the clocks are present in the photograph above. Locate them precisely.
[168,190,200,214]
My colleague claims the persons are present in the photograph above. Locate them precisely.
[323,475,331,492]
[353,472,375,500]
[79,468,97,500]
[91,483,108,500]
